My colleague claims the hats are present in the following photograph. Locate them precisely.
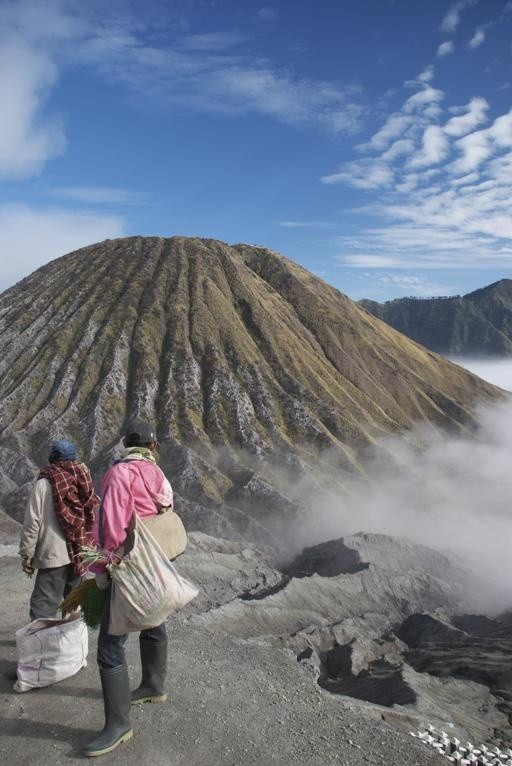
[51,440,78,462]
[125,421,158,443]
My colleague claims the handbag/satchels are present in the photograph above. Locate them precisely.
[13,610,89,692]
[133,507,186,561]
[107,516,200,637]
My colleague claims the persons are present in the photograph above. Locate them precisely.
[84,422,174,757]
[19,440,87,621]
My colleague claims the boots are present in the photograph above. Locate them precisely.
[130,638,168,705]
[84,665,135,757]
